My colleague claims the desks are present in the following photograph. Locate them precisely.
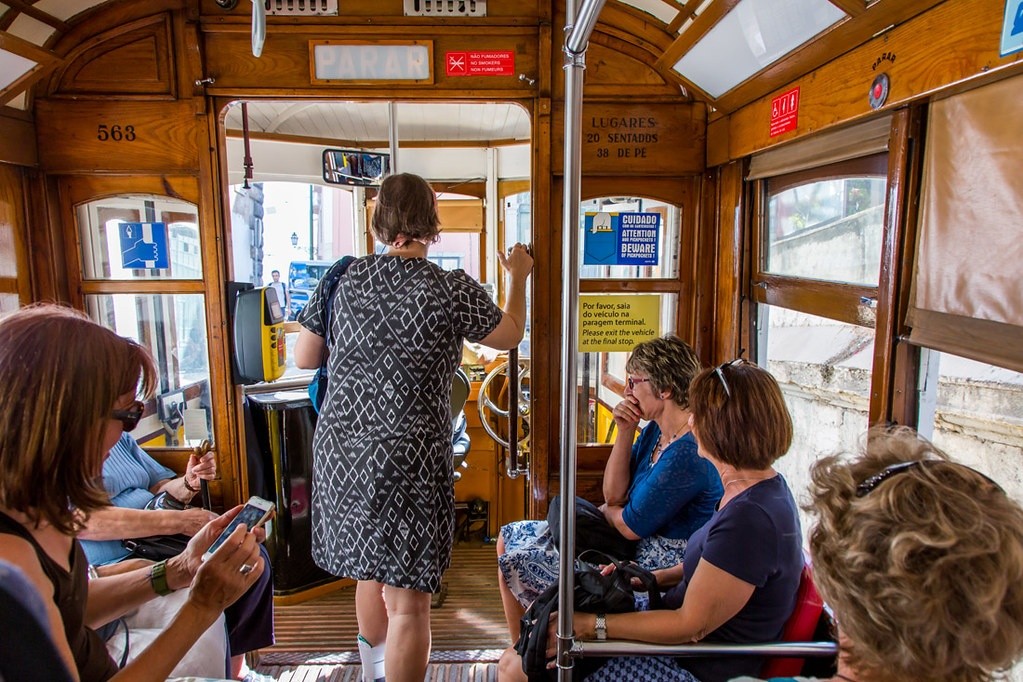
[246,388,360,603]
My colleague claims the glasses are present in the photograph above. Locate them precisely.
[854,459,1001,498]
[628,376,654,390]
[99,400,144,433]
[710,359,744,398]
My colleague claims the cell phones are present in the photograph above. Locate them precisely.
[201,495,275,562]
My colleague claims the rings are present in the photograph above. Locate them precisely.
[240,564,252,576]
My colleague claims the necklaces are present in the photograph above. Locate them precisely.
[658,422,688,455]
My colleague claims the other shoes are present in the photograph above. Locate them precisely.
[242,671,277,682]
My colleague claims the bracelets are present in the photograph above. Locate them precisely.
[150,557,176,596]
[183,475,200,493]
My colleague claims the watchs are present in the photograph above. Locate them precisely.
[594,612,607,640]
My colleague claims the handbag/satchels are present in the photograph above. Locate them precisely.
[514,548,660,682]
[547,494,640,564]
[308,256,356,414]
[121,491,192,562]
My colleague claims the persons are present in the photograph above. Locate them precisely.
[67,432,280,682]
[296,172,535,682]
[497,330,726,644]
[497,357,802,682]
[0,298,265,682]
[85,560,228,679]
[738,423,1022,682]
[268,270,291,318]
